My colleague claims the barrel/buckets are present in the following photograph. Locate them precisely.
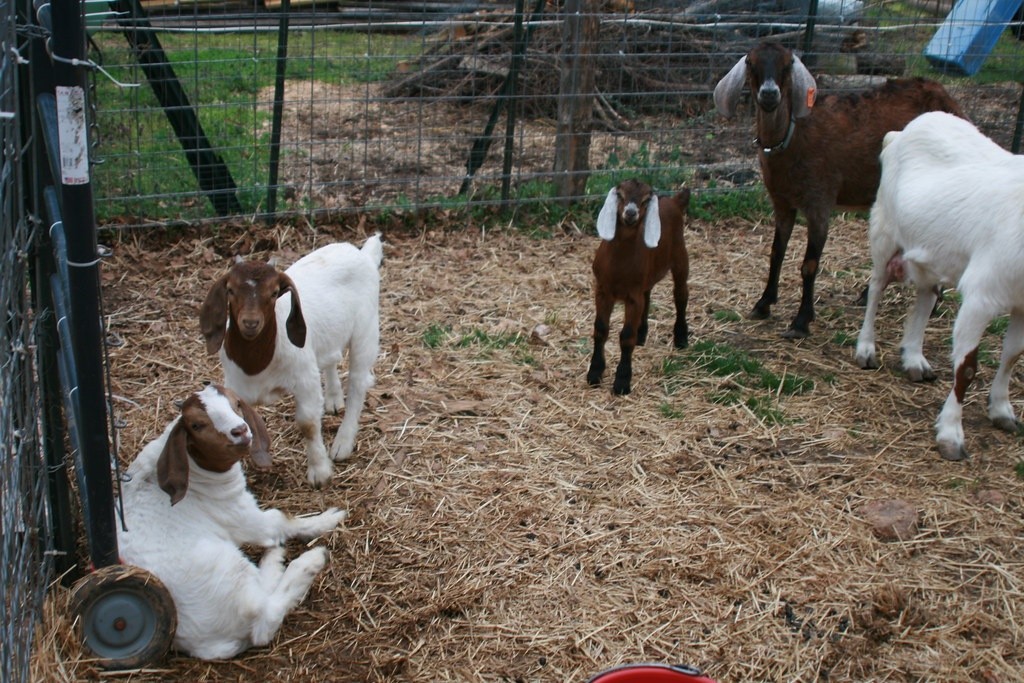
[587,663,717,683]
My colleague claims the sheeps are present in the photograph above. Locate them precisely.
[114,386,349,659]
[199,232,384,490]
[854,111,1024,461]
[713,42,975,339]
[587,179,690,395]
